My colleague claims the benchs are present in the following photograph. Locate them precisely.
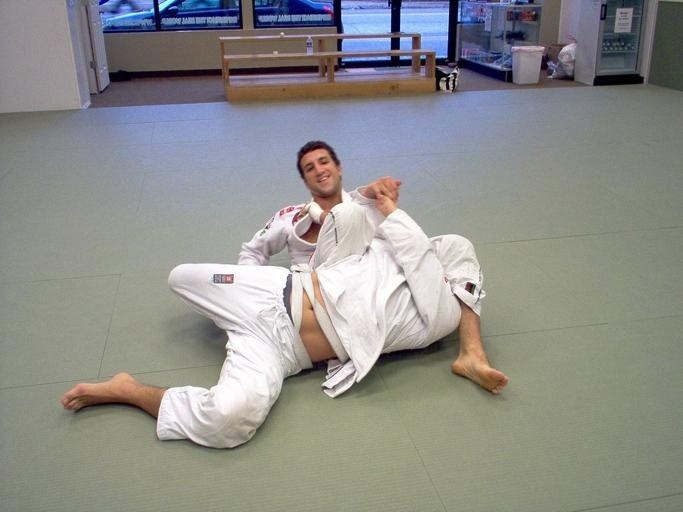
[223,49,437,101]
[218,32,421,81]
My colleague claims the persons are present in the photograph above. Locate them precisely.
[237,141,509,396]
[61,175,461,449]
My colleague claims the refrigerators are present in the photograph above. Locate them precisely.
[572,0,650,87]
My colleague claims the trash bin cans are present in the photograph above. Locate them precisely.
[512,46,545,85]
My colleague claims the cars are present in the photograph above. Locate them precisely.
[101,0,343,39]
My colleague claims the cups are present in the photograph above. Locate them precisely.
[506,10,521,20]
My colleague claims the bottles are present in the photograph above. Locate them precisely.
[601,40,635,51]
[306,36,313,56]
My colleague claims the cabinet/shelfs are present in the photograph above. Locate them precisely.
[454,0,543,82]
